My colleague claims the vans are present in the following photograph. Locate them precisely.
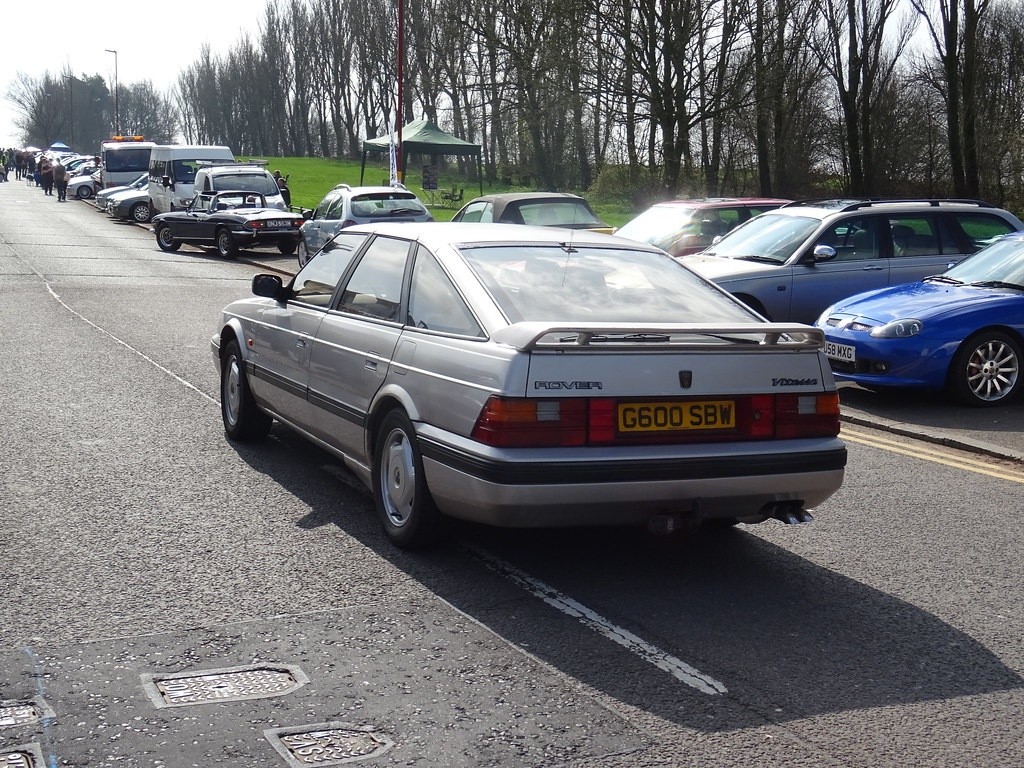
[148,144,235,217]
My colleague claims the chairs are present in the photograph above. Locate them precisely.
[216,203,227,210]
[728,220,743,231]
[891,223,916,257]
[698,220,721,245]
[825,229,838,247]
[846,230,875,259]
[440,183,465,210]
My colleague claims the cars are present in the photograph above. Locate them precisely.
[34,151,103,199]
[811,229,1023,408]
[151,189,304,261]
[193,158,289,214]
[103,181,152,223]
[208,222,846,550]
[448,191,620,237]
[296,183,432,269]
[95,171,149,208]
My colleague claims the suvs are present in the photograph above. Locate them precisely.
[584,195,806,274]
[604,197,1023,328]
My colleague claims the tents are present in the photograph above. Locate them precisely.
[25,146,41,152]
[360,119,484,201]
[50,141,70,151]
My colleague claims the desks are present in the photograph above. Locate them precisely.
[419,188,445,207]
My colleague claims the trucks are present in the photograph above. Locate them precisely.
[93,135,158,190]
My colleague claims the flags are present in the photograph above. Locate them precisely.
[387,121,398,188]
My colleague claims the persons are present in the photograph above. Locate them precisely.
[0,148,71,202]
[273,170,281,181]
[277,178,291,205]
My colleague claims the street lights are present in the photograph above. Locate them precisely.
[104,48,119,137]
[61,75,74,152]
